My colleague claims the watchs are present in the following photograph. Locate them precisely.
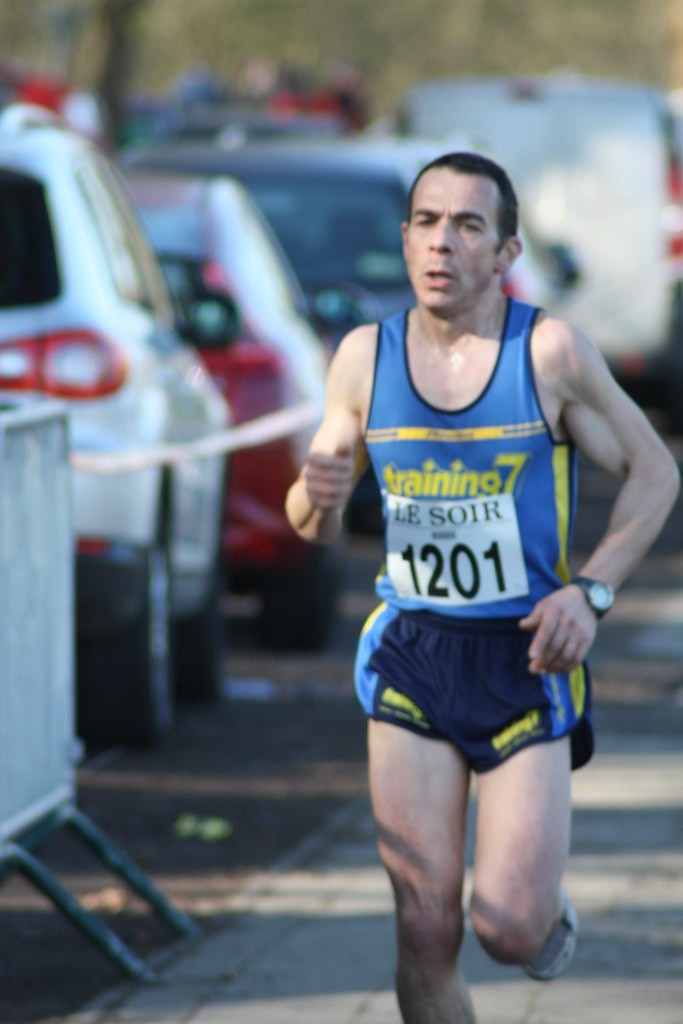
[571,577,615,620]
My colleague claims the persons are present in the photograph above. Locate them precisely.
[267,63,370,132]
[285,153,682,1024]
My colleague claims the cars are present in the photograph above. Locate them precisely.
[0,72,683,536]
[100,158,356,651]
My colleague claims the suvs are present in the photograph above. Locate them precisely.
[0,99,231,750]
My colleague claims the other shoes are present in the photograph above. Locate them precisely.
[524,897,579,980]
